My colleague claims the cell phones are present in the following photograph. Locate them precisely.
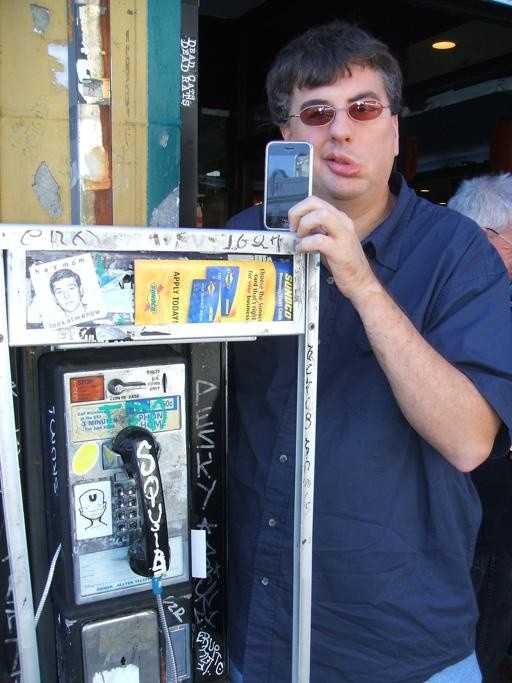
[261,141,314,233]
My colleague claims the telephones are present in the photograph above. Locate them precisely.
[37,345,193,607]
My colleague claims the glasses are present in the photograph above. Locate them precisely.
[283,98,394,128]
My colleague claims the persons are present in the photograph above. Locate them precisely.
[40,264,96,318]
[442,171,512,281]
[208,17,511,680]
